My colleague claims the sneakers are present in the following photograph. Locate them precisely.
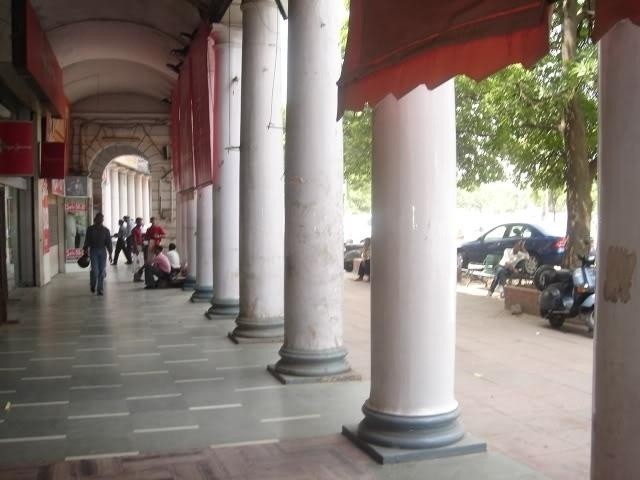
[98,289,103,295]
[91,288,95,292]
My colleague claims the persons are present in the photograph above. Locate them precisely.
[138,244,171,289]
[354,237,371,281]
[110,219,133,266]
[144,216,169,255]
[123,216,132,256]
[83,212,113,296]
[131,217,144,284]
[167,242,181,273]
[486,238,531,298]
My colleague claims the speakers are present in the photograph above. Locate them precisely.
[164,145,168,160]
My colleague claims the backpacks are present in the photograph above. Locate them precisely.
[127,235,134,252]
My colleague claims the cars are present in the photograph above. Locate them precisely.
[456,219,566,266]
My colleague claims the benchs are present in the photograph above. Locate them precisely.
[461,253,526,298]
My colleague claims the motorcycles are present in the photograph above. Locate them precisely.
[342,244,362,274]
[535,238,596,339]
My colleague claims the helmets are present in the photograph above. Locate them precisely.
[77,255,89,267]
